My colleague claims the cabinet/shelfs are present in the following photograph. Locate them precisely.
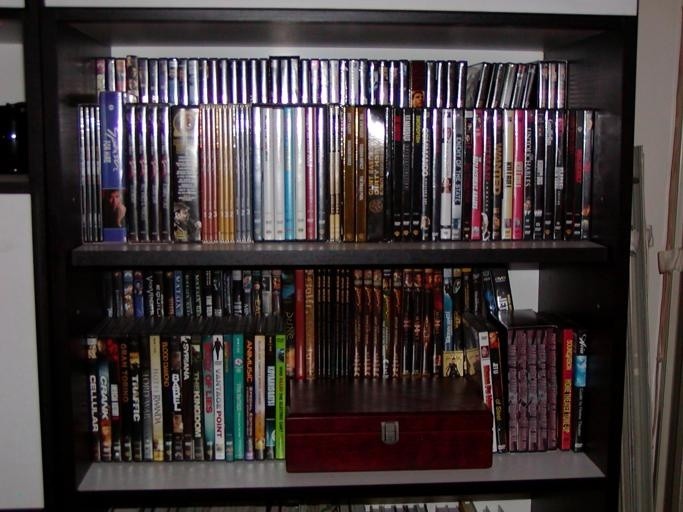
[20,0,638,511]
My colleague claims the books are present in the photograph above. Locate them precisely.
[77,55,622,247]
[81,266,590,462]
[103,500,532,512]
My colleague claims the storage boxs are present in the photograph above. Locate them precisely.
[285,375,494,473]
